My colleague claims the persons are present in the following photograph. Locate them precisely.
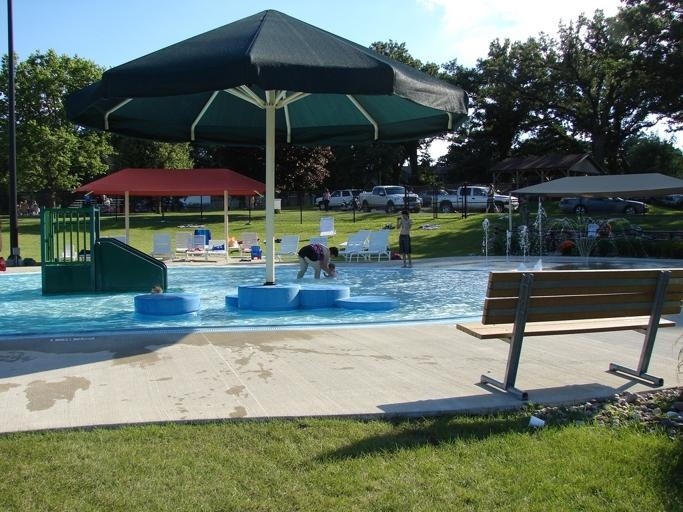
[297,243,338,278]
[85,195,90,208]
[323,188,331,211]
[17,199,41,216]
[486,184,499,213]
[397,210,411,268]
[323,263,336,277]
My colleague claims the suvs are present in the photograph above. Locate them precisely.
[315,189,365,210]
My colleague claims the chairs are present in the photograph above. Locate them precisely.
[113,236,127,243]
[338,228,390,262]
[207,240,227,262]
[148,234,175,262]
[228,240,242,257]
[174,232,193,253]
[274,235,300,262]
[309,236,328,248]
[243,231,262,253]
[319,217,336,240]
[186,235,207,262]
[60,242,80,261]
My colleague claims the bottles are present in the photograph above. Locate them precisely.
[0,257,6,271]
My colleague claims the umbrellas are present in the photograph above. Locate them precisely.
[61,11,469,285]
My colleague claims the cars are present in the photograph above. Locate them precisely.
[630,193,683,210]
[445,188,457,195]
[416,189,457,207]
[133,196,172,212]
[559,195,649,215]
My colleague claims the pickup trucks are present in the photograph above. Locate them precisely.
[430,185,519,214]
[358,185,421,214]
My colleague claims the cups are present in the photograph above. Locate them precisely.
[527,415,545,428]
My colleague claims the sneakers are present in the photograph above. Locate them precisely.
[400,264,413,268]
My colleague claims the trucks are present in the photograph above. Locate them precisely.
[177,196,210,206]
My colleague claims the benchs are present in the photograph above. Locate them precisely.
[457,267,681,402]
